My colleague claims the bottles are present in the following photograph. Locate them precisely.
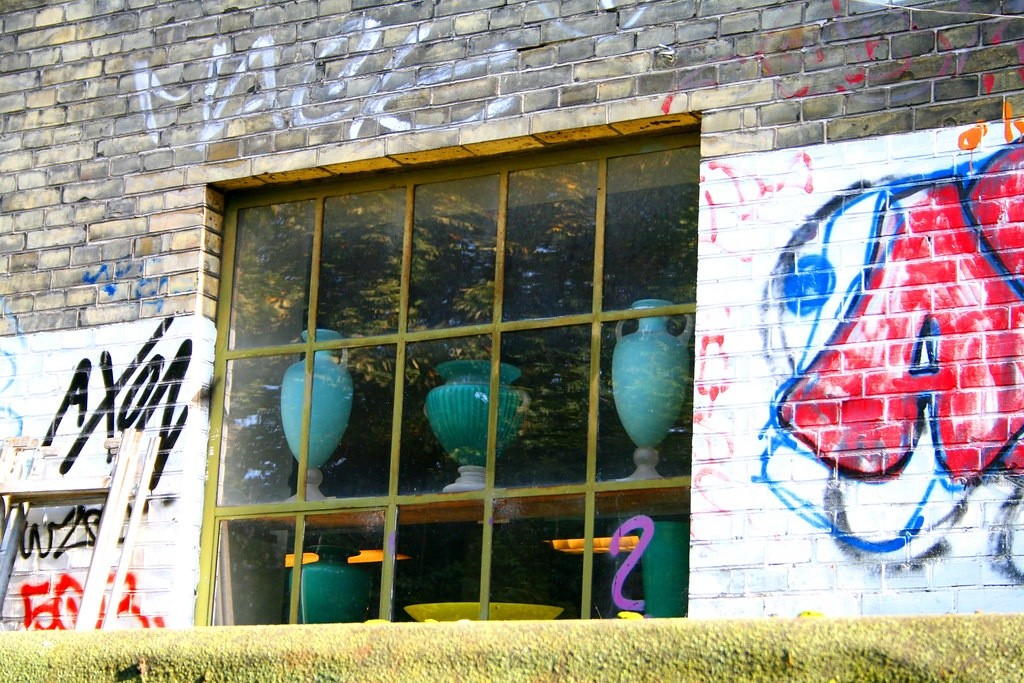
[425,359,532,466]
[616,298,693,446]
[288,545,373,624]
[281,329,354,469]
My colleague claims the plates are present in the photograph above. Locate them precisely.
[401,602,564,623]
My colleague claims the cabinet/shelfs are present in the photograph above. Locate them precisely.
[219,475,691,627]
